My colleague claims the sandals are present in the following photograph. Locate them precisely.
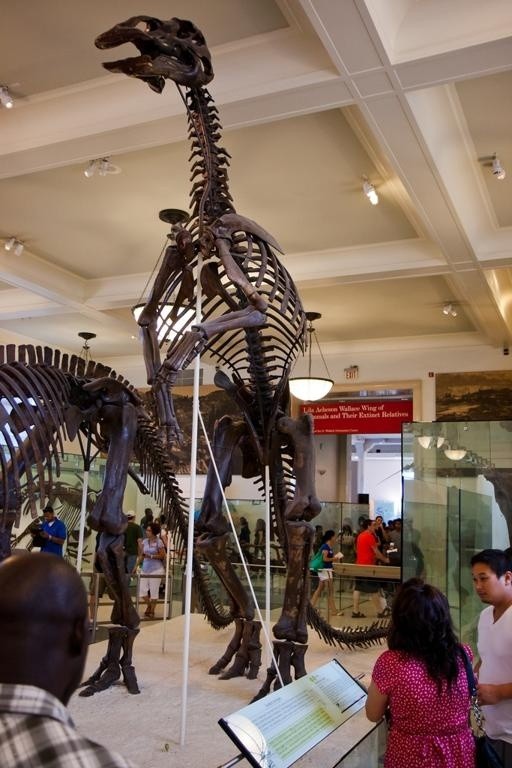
[352,611,366,618]
[378,609,391,618]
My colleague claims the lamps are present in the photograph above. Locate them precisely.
[4,236,26,256]
[490,151,504,179]
[414,422,471,466]
[130,208,206,341]
[443,302,458,319]
[85,158,112,176]
[360,175,380,209]
[1,86,14,110]
[287,312,335,403]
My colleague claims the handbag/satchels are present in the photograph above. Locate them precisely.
[308,552,325,571]
[470,705,487,739]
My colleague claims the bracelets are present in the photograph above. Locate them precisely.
[49,535,52,540]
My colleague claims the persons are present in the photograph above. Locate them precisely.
[365,579,477,768]
[40,507,67,554]
[309,515,403,618]
[0,552,128,768]
[223,516,266,579]
[470,549,511,765]
[123,507,169,618]
